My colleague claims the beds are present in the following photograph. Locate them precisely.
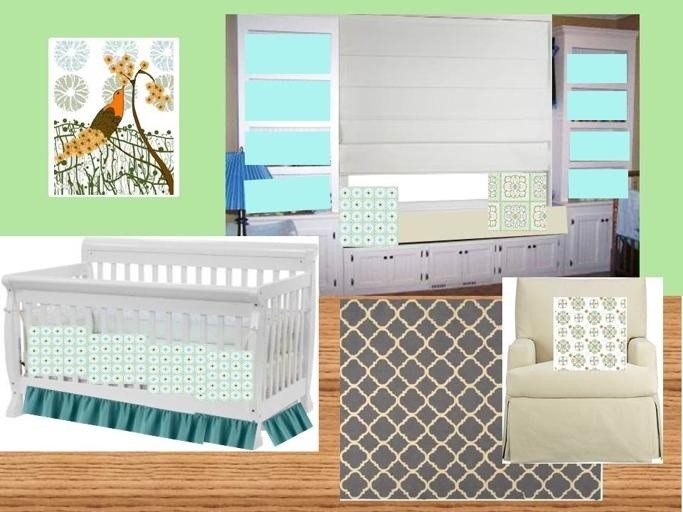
[0,237,317,451]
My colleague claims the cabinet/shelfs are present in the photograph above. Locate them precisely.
[225,12,643,296]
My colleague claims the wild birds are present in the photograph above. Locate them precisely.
[54,84,128,163]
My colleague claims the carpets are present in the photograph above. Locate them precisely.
[338,295,604,505]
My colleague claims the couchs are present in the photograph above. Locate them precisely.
[502,271,659,467]
